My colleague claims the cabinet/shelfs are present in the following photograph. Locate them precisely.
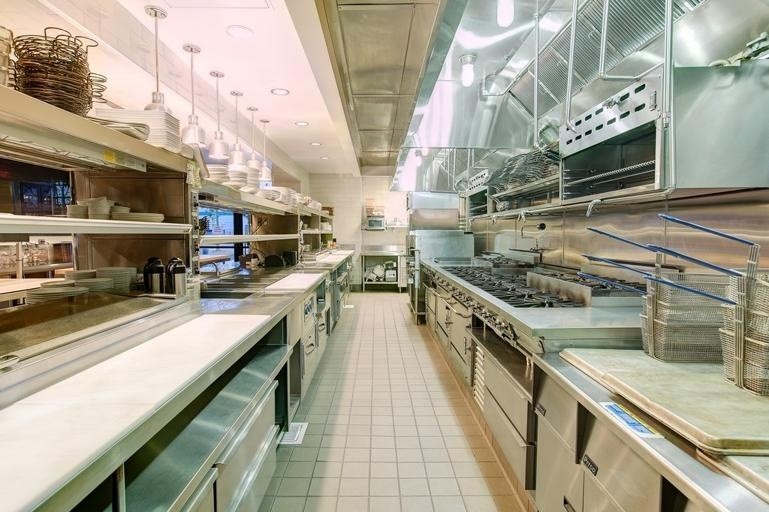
[361,225,404,294]
[401,264,718,512]
[2,86,355,510]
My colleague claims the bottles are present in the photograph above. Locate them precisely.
[143,254,187,297]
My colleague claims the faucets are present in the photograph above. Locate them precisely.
[200,261,221,278]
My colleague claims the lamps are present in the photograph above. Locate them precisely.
[496,0,514,28]
[460,64,474,88]
[142,5,272,184]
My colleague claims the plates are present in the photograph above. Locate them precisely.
[66,197,165,224]
[25,266,137,307]
[206,161,312,207]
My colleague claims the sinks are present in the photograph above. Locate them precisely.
[208,278,278,283]
[226,273,287,279]
[206,282,270,289]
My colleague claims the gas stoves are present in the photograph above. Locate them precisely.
[441,263,647,310]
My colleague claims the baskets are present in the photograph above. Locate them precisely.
[638,268,769,397]
